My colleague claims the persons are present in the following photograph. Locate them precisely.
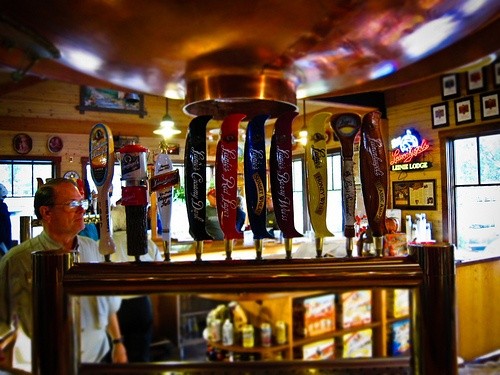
[206,189,224,240]
[0,178,128,375]
[235,197,246,232]
[146,205,163,236]
[97,206,164,362]
[266,193,279,230]
[0,184,12,257]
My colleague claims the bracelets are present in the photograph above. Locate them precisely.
[113,338,123,344]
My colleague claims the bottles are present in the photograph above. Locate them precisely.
[405,214,432,245]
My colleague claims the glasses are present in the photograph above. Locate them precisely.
[46,199,89,210]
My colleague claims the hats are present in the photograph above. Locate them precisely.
[0,184,8,200]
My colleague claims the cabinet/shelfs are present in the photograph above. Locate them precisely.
[198,284,412,362]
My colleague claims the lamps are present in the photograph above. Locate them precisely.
[295,100,310,147]
[152,97,182,139]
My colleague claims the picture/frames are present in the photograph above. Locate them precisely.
[431,103,450,128]
[74,83,148,119]
[48,136,64,153]
[440,74,460,99]
[492,61,500,87]
[465,66,488,96]
[13,133,33,154]
[479,91,500,120]
[455,97,477,125]
